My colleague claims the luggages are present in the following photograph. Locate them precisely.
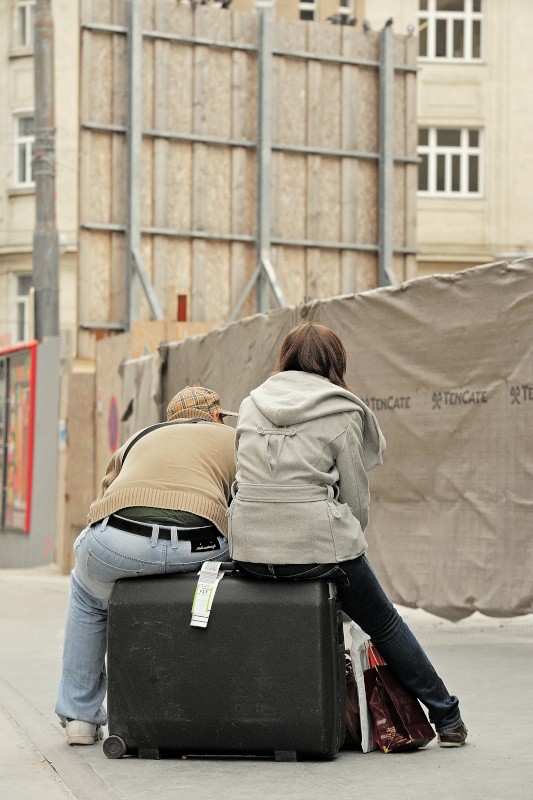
[103,571,346,762]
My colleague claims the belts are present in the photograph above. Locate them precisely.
[102,514,224,541]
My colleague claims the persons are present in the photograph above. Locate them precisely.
[55,386,238,746]
[224,320,467,748]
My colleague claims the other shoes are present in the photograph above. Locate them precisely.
[64,719,104,744]
[438,724,468,746]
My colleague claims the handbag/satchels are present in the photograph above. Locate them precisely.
[342,649,360,744]
[349,624,378,753]
[363,639,436,753]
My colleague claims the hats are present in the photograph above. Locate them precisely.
[167,386,240,417]
